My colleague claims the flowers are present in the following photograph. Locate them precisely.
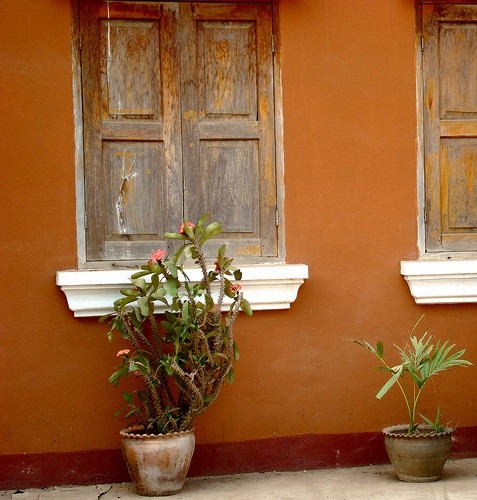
[97,210,254,435]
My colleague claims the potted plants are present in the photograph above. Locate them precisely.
[345,312,475,483]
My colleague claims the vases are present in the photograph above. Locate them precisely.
[120,424,196,497]
